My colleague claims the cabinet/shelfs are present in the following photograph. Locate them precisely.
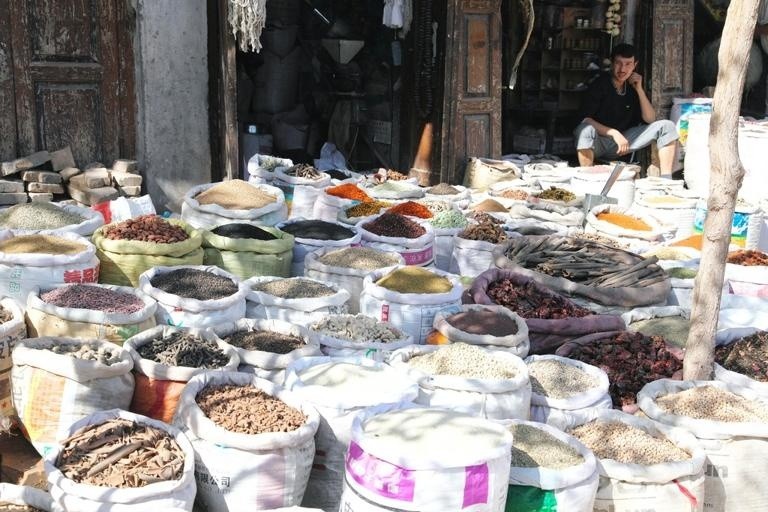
[502,0,612,168]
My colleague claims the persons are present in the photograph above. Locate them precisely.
[573,43,678,181]
